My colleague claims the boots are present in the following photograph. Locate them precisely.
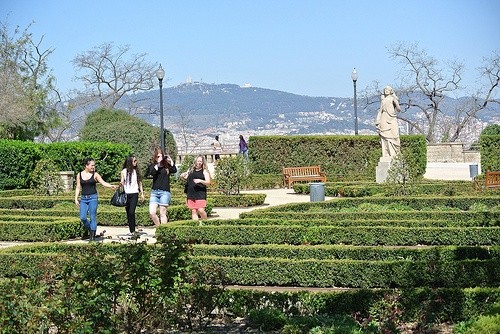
[90,230,96,242]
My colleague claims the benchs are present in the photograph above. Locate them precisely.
[283,165,327,189]
[485,171,500,189]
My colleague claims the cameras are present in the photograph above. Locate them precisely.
[162,156,167,159]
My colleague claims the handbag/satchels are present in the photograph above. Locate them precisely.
[111,185,127,207]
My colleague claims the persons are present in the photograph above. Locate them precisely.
[180,154,210,220]
[211,136,222,160]
[119,153,144,236]
[375,86,401,156]
[144,147,177,226]
[239,135,248,160]
[75,157,116,241]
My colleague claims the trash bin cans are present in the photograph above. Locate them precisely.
[469,164,479,177]
[309,182,325,202]
